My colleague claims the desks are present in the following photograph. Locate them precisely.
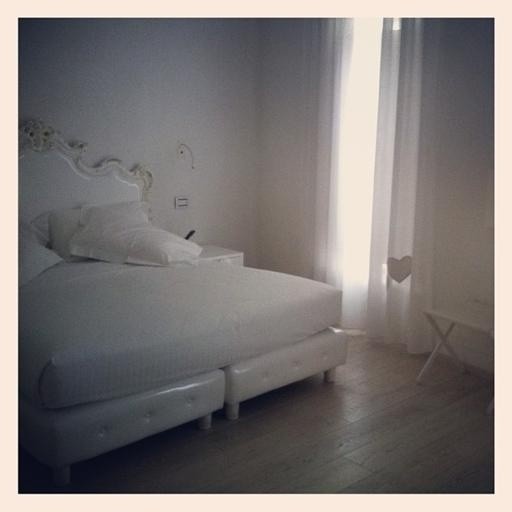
[413,301,494,385]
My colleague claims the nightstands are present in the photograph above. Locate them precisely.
[198,245,244,266]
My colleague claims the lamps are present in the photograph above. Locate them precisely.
[176,136,195,173]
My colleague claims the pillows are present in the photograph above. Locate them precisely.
[18,232,61,290]
[30,197,153,263]
[69,203,203,267]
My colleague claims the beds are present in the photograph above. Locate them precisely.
[18,115,349,481]
